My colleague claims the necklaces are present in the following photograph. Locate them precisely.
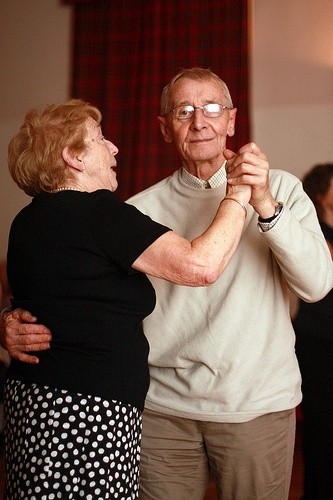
[50,186,82,193]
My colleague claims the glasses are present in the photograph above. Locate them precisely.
[161,103,233,120]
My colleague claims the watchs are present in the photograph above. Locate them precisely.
[258,200,284,224]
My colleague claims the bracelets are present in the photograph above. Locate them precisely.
[220,196,248,220]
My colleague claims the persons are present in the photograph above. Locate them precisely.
[0,100,252,500]
[0,66,333,500]
[285,161,333,500]
